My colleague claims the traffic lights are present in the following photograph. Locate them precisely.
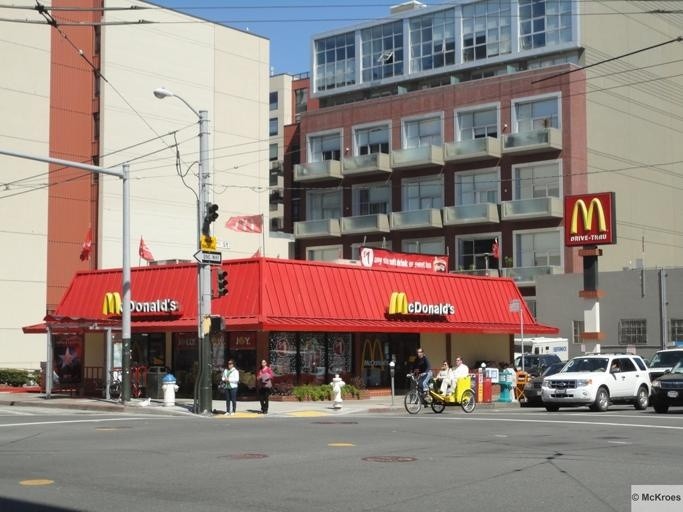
[208,315,225,333]
[217,267,229,298]
[204,199,219,223]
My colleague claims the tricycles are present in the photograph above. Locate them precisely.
[401,372,478,414]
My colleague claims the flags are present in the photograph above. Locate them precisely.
[225,214,262,233]
[139,238,157,262]
[80,227,93,264]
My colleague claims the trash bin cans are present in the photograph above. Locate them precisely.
[147,367,168,399]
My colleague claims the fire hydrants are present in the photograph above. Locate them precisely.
[158,372,180,408]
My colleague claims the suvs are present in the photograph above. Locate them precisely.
[512,347,682,413]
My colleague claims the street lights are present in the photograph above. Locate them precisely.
[151,83,219,414]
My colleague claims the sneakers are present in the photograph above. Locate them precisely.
[258,410,267,414]
[224,412,235,415]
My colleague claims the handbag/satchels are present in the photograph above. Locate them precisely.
[218,382,226,393]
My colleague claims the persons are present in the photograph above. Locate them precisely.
[410,347,434,393]
[432,360,453,384]
[256,358,275,415]
[222,358,240,418]
[440,355,470,398]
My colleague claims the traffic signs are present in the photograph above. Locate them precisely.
[190,247,222,266]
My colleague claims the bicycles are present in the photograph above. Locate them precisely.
[105,365,122,402]
